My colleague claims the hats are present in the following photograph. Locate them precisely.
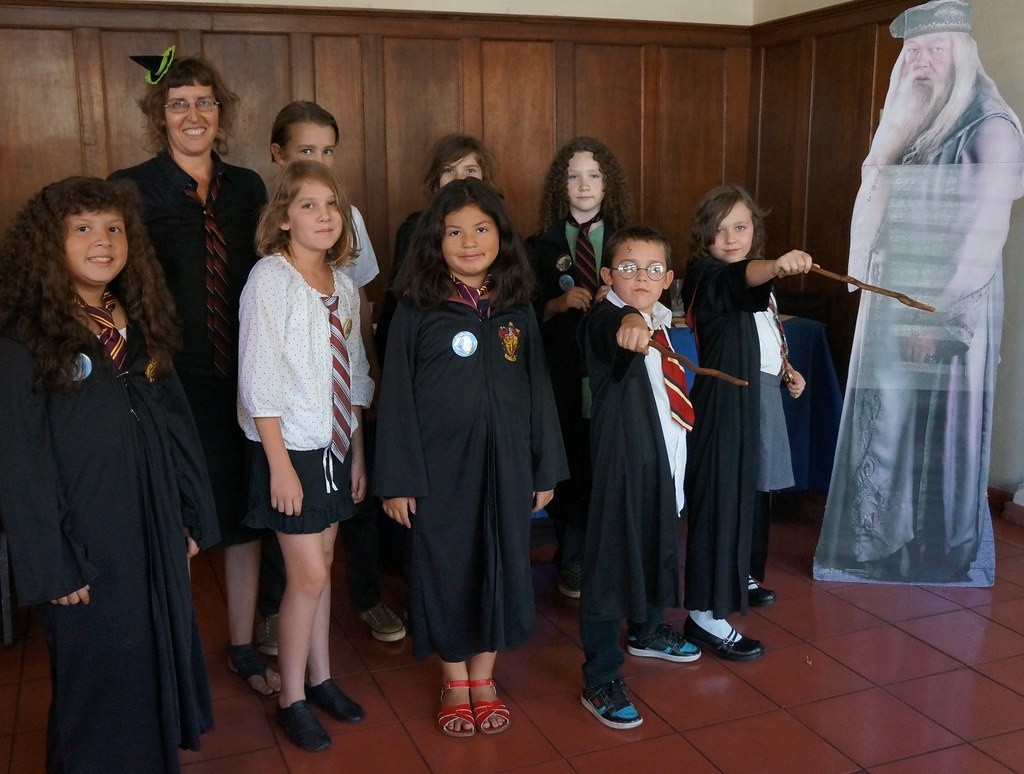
[129,45,176,85]
[889,1,973,39]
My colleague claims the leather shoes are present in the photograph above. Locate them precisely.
[276,699,331,752]
[305,677,366,723]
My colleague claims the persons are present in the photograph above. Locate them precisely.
[389,177,569,736]
[110,45,283,698]
[678,184,820,660]
[820,0,1023,586]
[391,136,634,598]
[0,177,221,774]
[236,160,376,753]
[580,225,702,728]
[256,101,404,655]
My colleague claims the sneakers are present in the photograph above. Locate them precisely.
[256,613,279,656]
[624,622,702,662]
[359,596,406,642]
[557,558,581,597]
[580,678,643,729]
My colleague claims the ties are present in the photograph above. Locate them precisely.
[322,296,352,463]
[768,294,784,343]
[72,290,129,373]
[567,210,603,304]
[182,178,233,384]
[647,325,695,432]
[449,272,495,309]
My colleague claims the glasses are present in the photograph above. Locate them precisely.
[162,98,220,113]
[611,260,667,281]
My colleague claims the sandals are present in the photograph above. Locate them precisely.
[226,639,281,698]
[437,679,513,737]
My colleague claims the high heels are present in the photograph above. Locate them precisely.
[748,576,776,607]
[684,614,766,662]
